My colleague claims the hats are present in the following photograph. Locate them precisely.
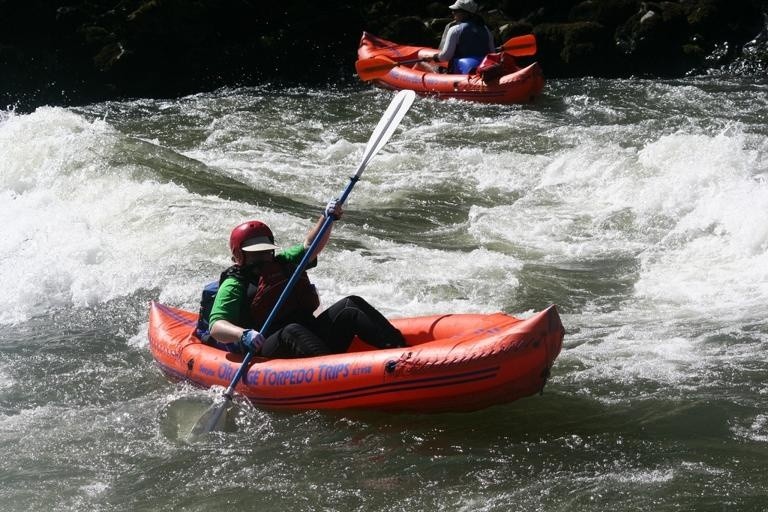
[449,0,478,14]
[241,236,278,252]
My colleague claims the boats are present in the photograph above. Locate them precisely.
[357,32,544,105]
[147,299,565,416]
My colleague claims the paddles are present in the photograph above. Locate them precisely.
[356,34,536,82]
[188,89,416,436]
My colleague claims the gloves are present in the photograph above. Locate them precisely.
[322,199,343,220]
[422,55,433,63]
[240,329,265,353]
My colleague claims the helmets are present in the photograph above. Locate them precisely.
[230,220,275,265]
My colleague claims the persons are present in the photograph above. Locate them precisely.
[206,194,412,359]
[417,0,496,76]
